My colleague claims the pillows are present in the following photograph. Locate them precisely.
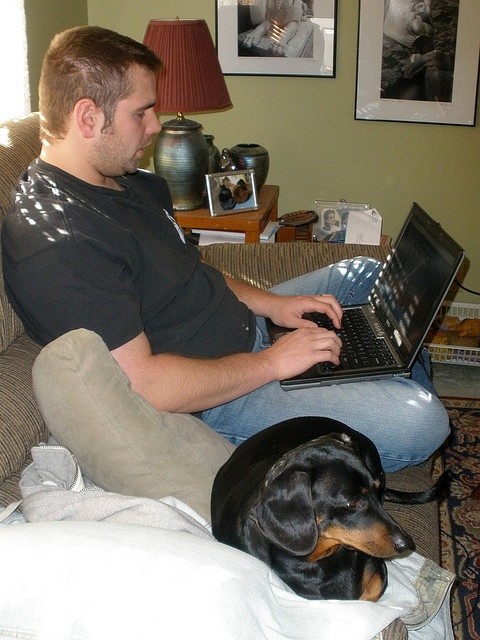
[30,327,241,535]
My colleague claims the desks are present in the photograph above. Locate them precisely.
[172,185,280,243]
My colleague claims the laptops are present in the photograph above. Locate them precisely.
[263,201,469,391]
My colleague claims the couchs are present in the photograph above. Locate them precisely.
[0,111,440,639]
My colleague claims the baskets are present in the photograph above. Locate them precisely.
[423,301,480,367]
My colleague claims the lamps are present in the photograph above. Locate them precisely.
[141,16,236,213]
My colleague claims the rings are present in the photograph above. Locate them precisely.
[320,294,322,297]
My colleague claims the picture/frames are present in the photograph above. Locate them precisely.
[314,200,372,242]
[214,0,335,76]
[205,166,259,217]
[353,0,478,125]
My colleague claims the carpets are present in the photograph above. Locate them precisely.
[431,395,480,638]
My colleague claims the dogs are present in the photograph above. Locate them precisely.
[207,411,418,605]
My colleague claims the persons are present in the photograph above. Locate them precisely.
[0,25,451,471]
[322,209,340,234]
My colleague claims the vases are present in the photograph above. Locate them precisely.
[204,134,221,175]
[228,143,270,194]
